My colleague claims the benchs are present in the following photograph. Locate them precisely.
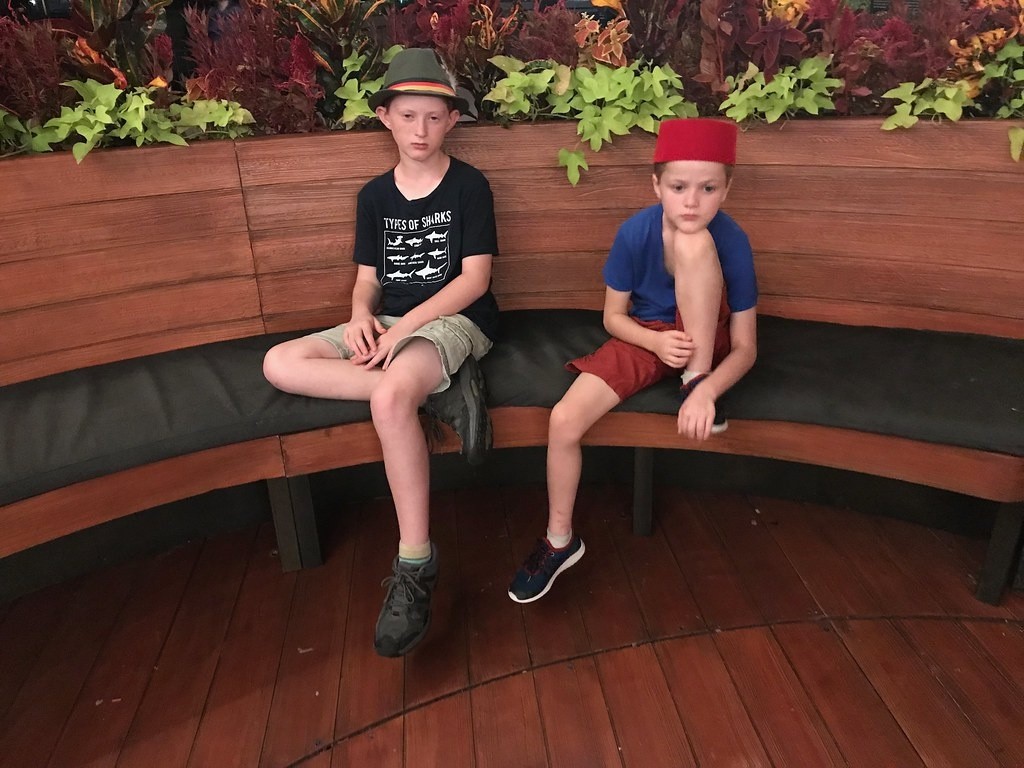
[2,116,1023,607]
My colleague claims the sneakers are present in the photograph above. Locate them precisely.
[679,374,728,433]
[508,532,585,603]
[428,357,494,472]
[373,543,440,657]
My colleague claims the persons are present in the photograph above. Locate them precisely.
[256,44,505,663]
[502,113,764,611]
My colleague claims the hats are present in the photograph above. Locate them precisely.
[368,48,469,116]
[654,119,737,166]
